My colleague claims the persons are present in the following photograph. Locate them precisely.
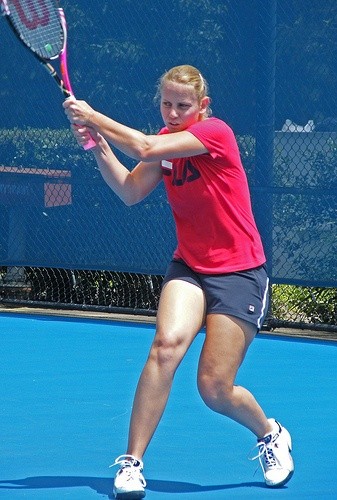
[63,65,294,500]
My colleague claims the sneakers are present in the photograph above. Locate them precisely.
[247,418,295,489]
[107,454,146,500]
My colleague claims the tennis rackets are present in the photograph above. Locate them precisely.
[1,0,98,153]
